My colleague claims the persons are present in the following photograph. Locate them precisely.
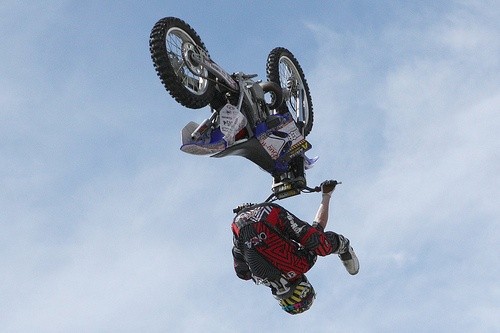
[231,179,359,314]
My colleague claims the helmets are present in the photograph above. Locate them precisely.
[279,281,314,315]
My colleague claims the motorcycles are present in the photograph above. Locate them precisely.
[150,16,342,213]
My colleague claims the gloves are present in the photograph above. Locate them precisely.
[321,179,337,195]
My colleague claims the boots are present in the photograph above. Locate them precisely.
[335,233,359,275]
